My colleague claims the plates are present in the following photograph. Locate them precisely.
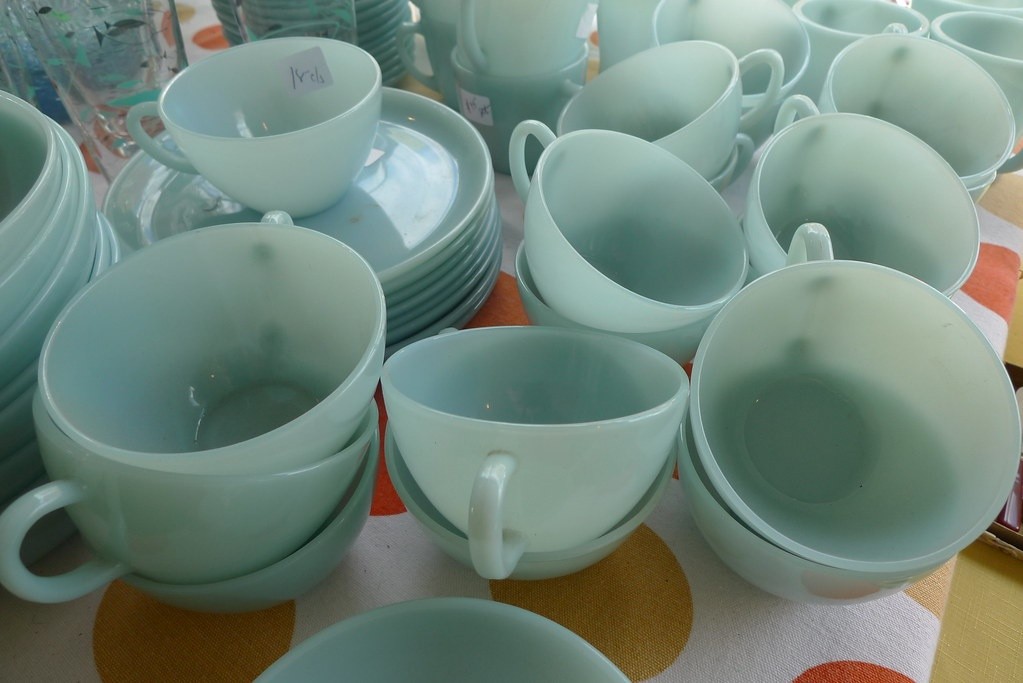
[0,92,126,508]
[99,85,504,357]
[208,0,413,85]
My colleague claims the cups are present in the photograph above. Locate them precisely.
[377,0,1023,603]
[0,210,389,611]
[250,596,632,683]
[0,0,357,187]
